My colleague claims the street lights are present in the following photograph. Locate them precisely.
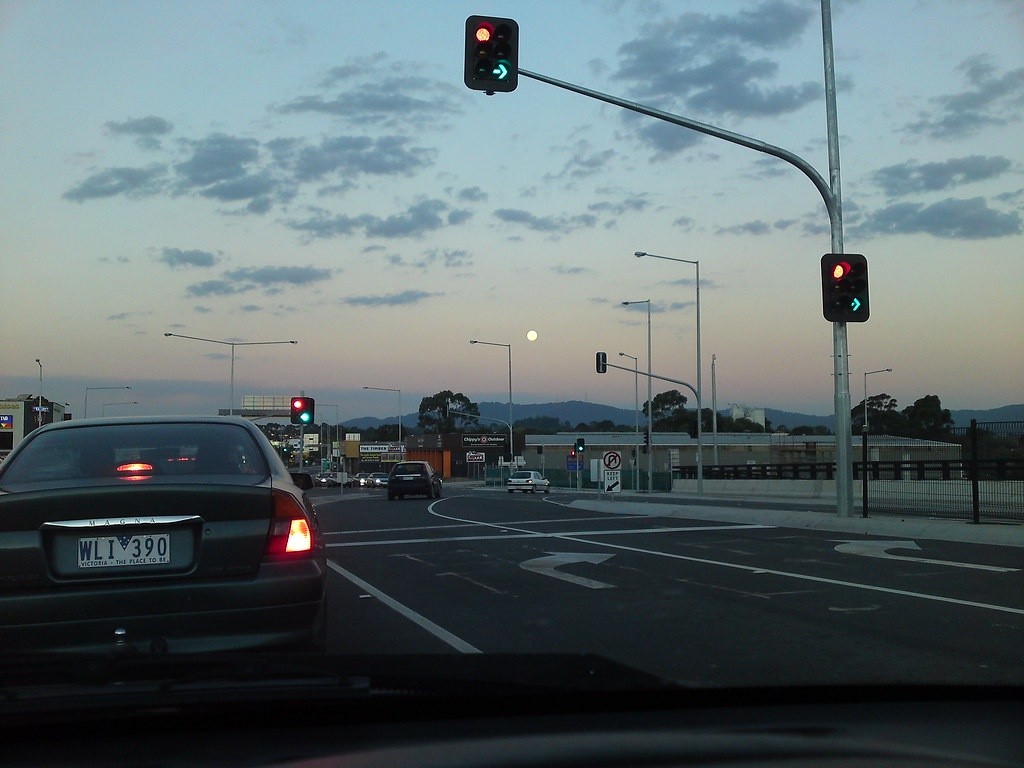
[35,358,43,427]
[84,386,133,419]
[363,386,402,462]
[621,299,652,495]
[469,340,513,477]
[619,352,639,492]
[164,332,298,416]
[102,401,138,418]
[864,368,893,424]
[634,251,704,498]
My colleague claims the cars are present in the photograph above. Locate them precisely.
[507,470,550,494]
[0,416,329,742]
[387,460,441,501]
[314,472,352,488]
[367,473,389,489]
[353,473,368,487]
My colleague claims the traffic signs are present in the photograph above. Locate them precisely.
[604,470,621,493]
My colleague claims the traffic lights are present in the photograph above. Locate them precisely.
[290,396,315,424]
[643,430,649,446]
[821,252,870,322]
[462,14,521,96]
[571,450,575,457]
[577,438,585,452]
[631,448,635,457]
